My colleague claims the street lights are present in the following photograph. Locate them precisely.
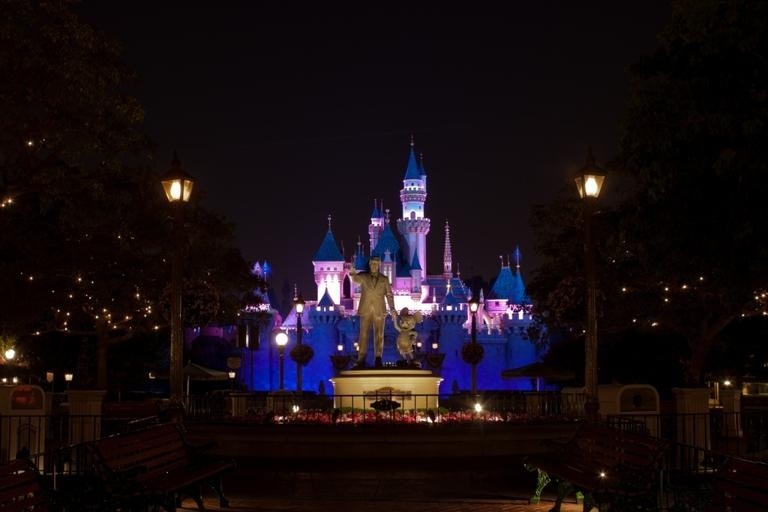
[292,293,306,395]
[467,293,481,395]
[273,331,291,392]
[157,166,196,415]
[568,153,613,428]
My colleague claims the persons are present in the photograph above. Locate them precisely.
[349,256,396,370]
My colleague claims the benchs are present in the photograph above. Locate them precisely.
[521,423,671,512]
[703,457,768,512]
[69,423,235,512]
[0,457,64,512]
